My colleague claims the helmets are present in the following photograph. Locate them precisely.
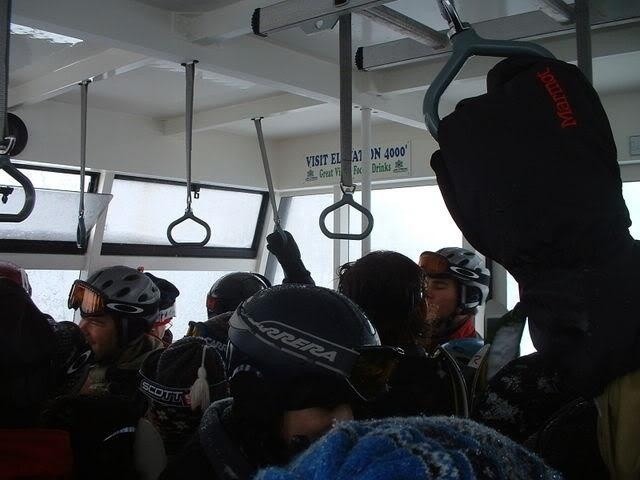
[226,283,382,411]
[206,272,272,319]
[436,246,491,315]
[85,266,160,333]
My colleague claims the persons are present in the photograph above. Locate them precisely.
[429,55,639,480]
[1,231,562,480]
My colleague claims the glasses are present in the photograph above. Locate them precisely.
[67,279,108,318]
[164,322,173,331]
[349,344,404,411]
[419,251,451,275]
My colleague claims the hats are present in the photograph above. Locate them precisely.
[144,272,180,323]
[254,416,563,480]
[137,336,228,412]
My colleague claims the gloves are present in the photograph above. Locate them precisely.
[430,54,640,394]
[266,231,310,279]
